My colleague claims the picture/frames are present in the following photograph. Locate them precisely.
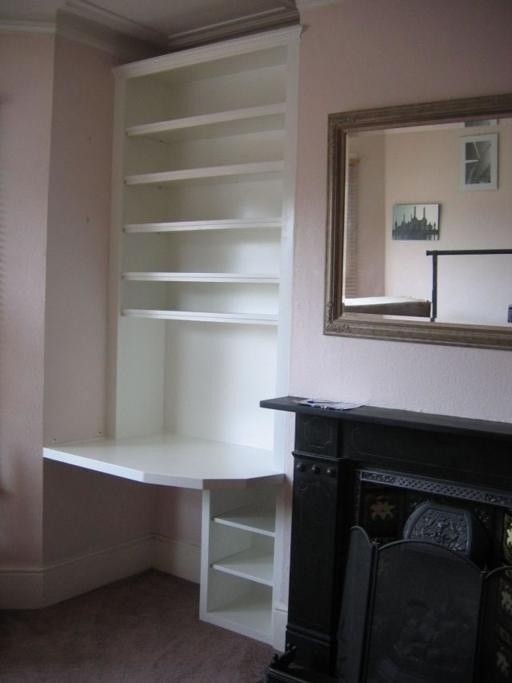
[460,118,498,128]
[457,132,499,192]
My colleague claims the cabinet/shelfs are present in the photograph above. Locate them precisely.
[41,23,307,652]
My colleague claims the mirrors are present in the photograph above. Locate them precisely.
[321,94,512,350]
